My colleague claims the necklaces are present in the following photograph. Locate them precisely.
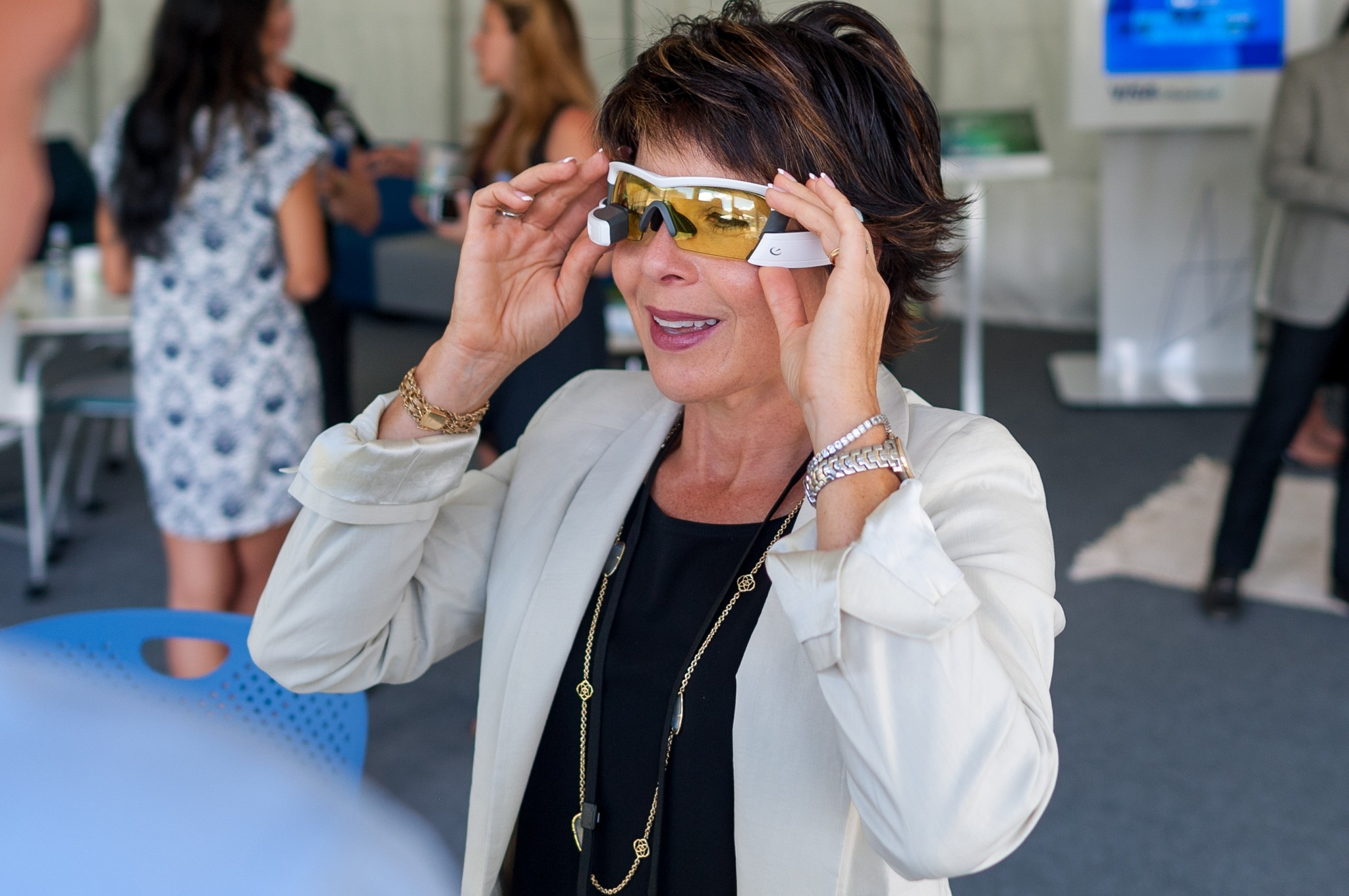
[573,490,807,894]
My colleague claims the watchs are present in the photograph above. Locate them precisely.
[801,433,915,509]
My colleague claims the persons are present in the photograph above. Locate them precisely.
[1190,0,1349,619]
[0,0,602,687]
[246,0,1065,896]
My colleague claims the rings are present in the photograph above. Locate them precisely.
[497,207,525,219]
[829,239,869,266]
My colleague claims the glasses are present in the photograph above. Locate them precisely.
[586,159,865,269]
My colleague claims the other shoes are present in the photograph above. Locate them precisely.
[1201,571,1239,621]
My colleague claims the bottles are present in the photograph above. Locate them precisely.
[48,222,73,319]
[327,108,355,171]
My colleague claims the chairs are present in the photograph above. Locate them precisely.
[0,609,369,790]
[24,137,100,257]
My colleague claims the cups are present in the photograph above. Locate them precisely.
[72,245,104,316]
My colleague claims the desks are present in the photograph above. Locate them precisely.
[0,246,150,584]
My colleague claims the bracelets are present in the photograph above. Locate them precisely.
[806,414,892,473]
[398,367,489,433]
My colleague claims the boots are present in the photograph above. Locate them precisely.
[1288,392,1343,467]
